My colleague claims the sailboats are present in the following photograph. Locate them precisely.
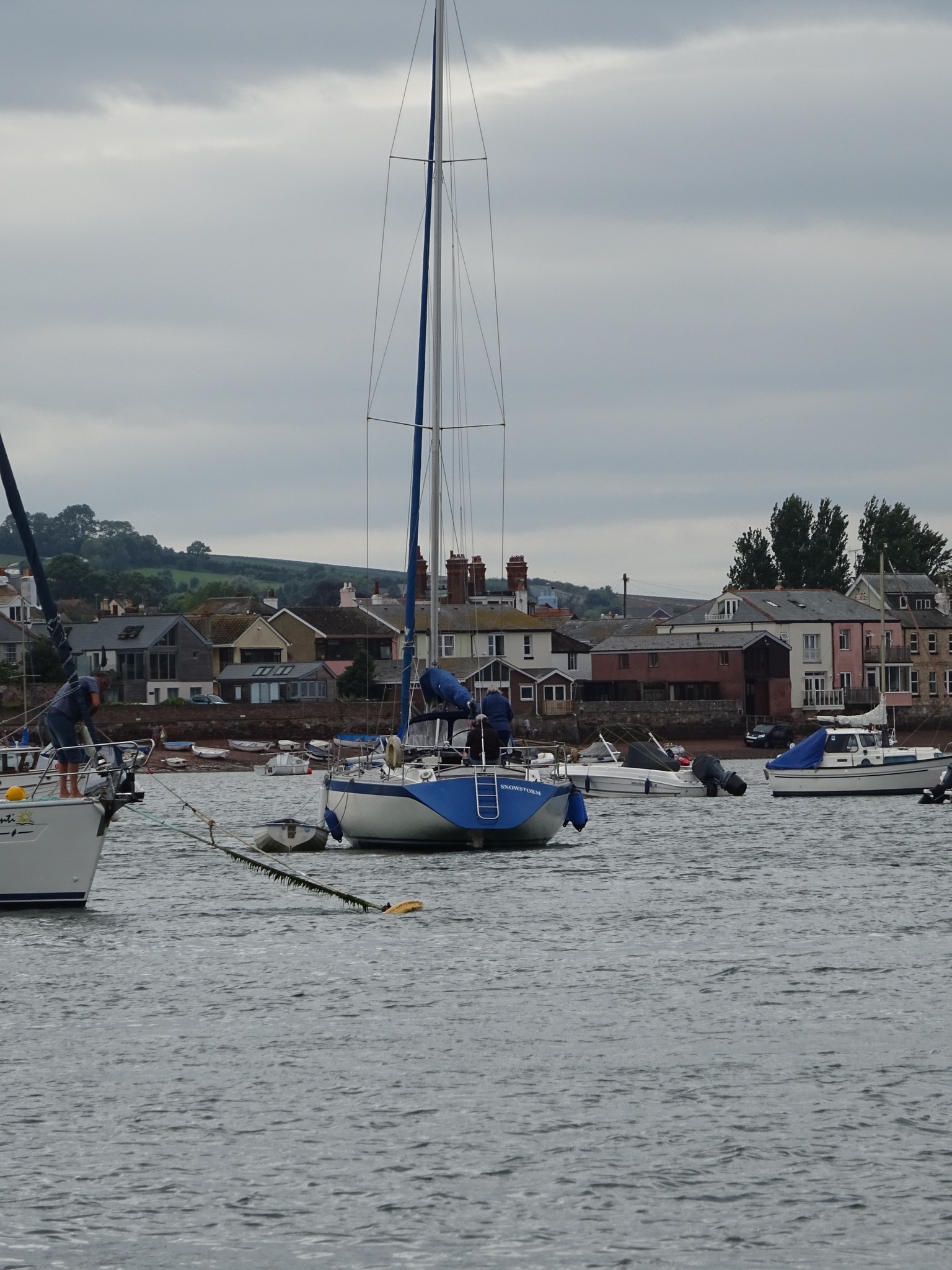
[0,432,157,910]
[324,0,576,850]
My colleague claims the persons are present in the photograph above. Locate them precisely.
[478,684,515,766]
[45,671,111,798]
[464,714,505,766]
[151,725,167,750]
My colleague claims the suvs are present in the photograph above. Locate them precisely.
[188,694,233,705]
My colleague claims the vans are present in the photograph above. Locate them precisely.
[743,722,793,749]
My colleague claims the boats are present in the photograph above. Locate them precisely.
[309,739,332,752]
[253,751,313,776]
[162,741,196,751]
[191,745,230,760]
[303,742,333,762]
[550,723,747,797]
[228,739,272,752]
[258,741,277,748]
[763,544,952,804]
[278,740,300,751]
[0,597,149,802]
[333,732,386,750]
[165,757,188,767]
